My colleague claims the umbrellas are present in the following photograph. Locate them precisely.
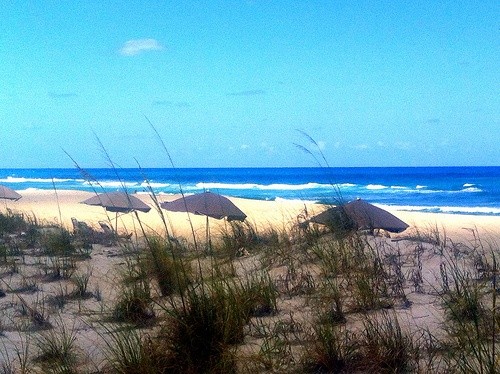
[309,197,409,232]
[0,185,22,201]
[78,190,151,213]
[160,190,247,222]
